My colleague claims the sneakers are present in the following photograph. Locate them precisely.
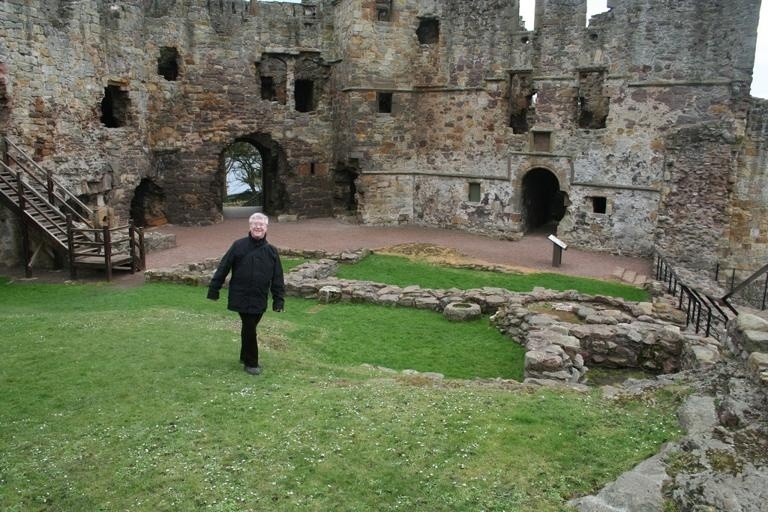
[244,365,261,375]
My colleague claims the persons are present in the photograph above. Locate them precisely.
[206,212,285,376]
